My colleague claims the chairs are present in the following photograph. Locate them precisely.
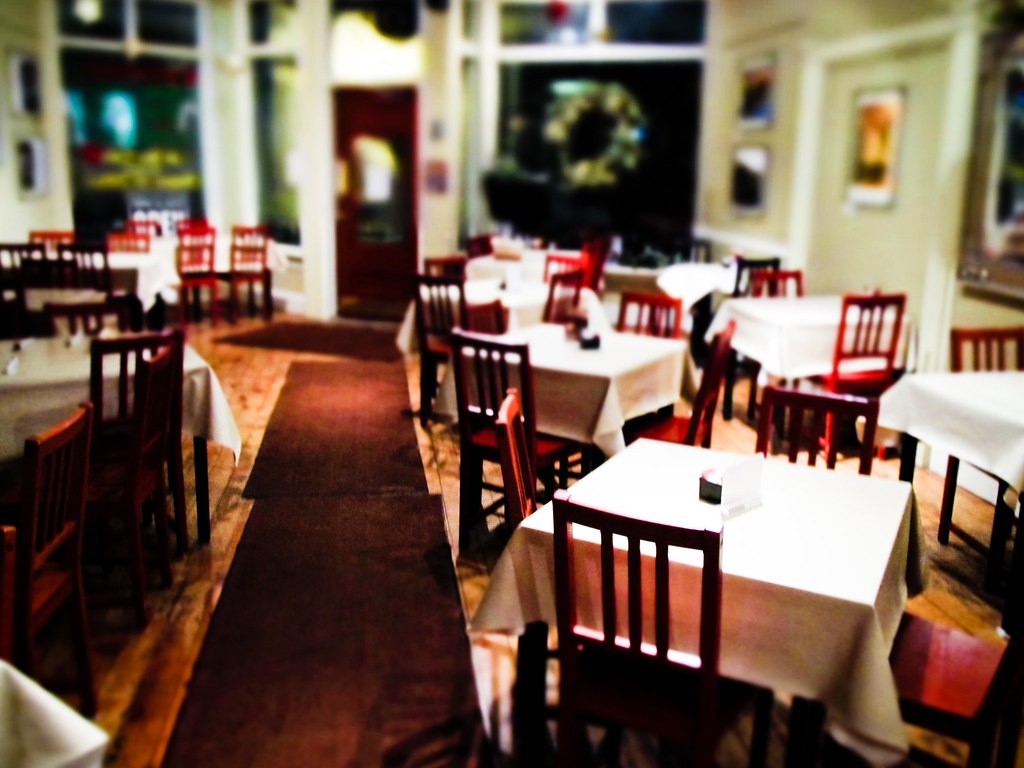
[0,218,1024,768]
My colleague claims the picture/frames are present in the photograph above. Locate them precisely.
[733,47,781,133]
[844,81,908,211]
[727,140,774,223]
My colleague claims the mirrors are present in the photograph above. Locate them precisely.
[955,0,1024,305]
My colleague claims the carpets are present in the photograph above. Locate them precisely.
[213,320,402,363]
[240,359,430,500]
[161,494,489,768]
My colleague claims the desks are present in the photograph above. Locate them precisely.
[431,323,701,471]
[469,439,931,768]
[654,260,749,334]
[132,232,290,319]
[465,248,584,281]
[704,293,919,456]
[0,337,241,634]
[858,369,1024,596]
[0,250,183,333]
[395,278,608,419]
